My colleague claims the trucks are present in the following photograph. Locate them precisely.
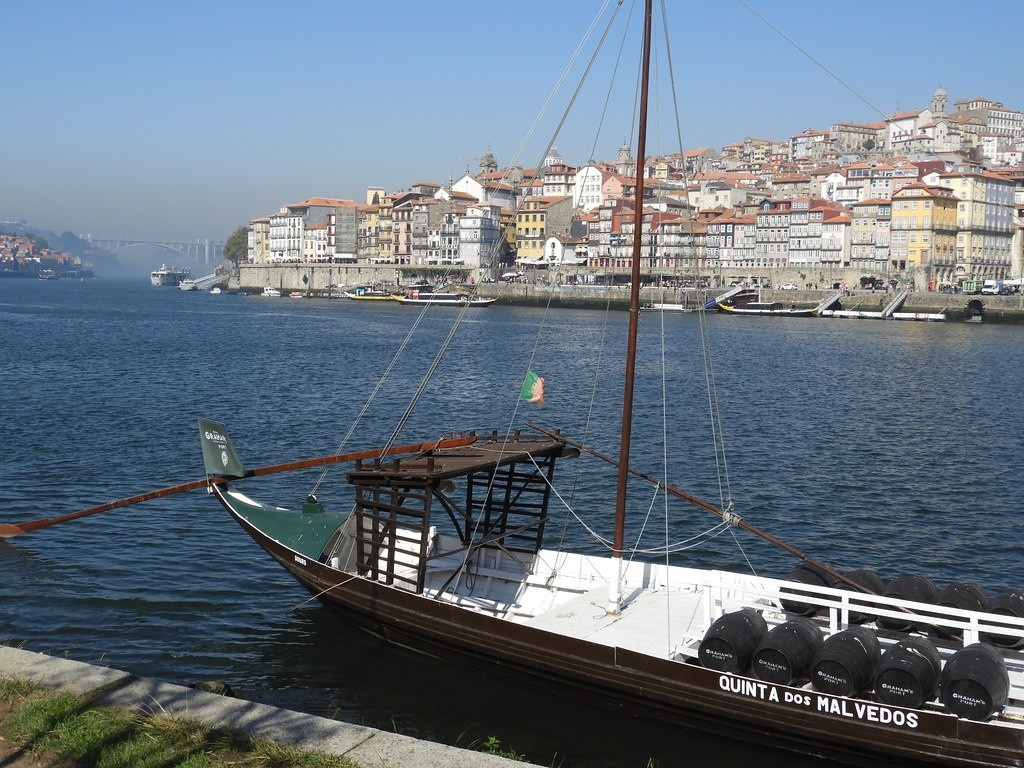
[981,279,1003,295]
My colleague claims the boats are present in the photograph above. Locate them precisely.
[260,286,281,297]
[289,292,303,298]
[716,291,820,316]
[39,269,59,280]
[150,265,188,287]
[342,286,398,300]
[209,287,224,295]
[390,285,500,307]
[177,279,195,292]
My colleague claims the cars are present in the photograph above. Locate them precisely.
[779,284,799,290]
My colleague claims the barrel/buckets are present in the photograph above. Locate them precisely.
[986,591,1024,648]
[940,642,1010,721]
[752,617,823,687]
[828,569,883,623]
[810,626,880,697]
[698,609,768,675]
[934,583,991,637]
[872,636,941,709]
[876,575,937,632]
[779,559,834,616]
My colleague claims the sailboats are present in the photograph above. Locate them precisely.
[198,1,1024,768]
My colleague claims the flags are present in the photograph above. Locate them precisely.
[519,370,545,404]
